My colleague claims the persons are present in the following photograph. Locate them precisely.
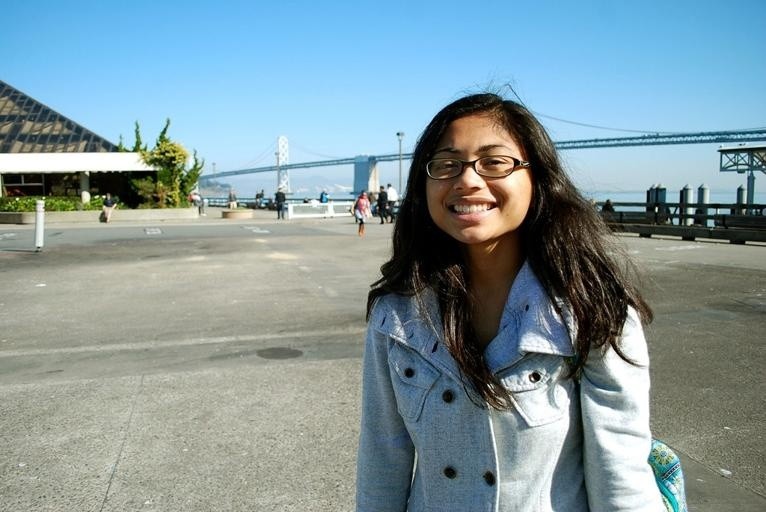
[321,189,328,203]
[189,193,204,215]
[230,191,237,209]
[351,183,398,236]
[589,199,615,232]
[304,198,309,203]
[355,77,670,512]
[276,188,286,219]
[259,190,264,210]
[102,192,117,222]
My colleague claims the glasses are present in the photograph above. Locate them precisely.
[425,155,531,178]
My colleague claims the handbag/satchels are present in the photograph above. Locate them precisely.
[648,438,688,512]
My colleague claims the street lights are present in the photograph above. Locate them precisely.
[396,131,405,194]
[274,151,282,186]
[211,162,217,192]
